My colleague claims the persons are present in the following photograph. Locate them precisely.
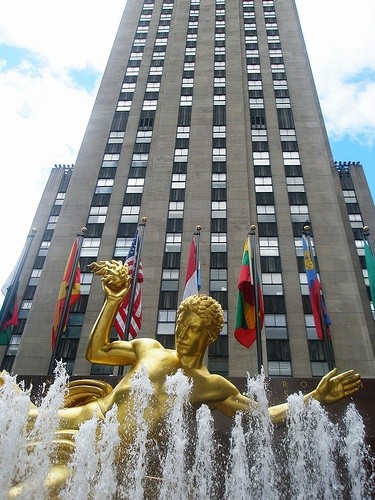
[1,260,362,500]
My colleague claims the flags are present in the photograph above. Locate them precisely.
[113,227,142,341]
[362,237,375,306]
[0,280,19,346]
[52,238,82,352]
[234,236,265,349]
[302,234,332,341]
[182,237,201,300]
